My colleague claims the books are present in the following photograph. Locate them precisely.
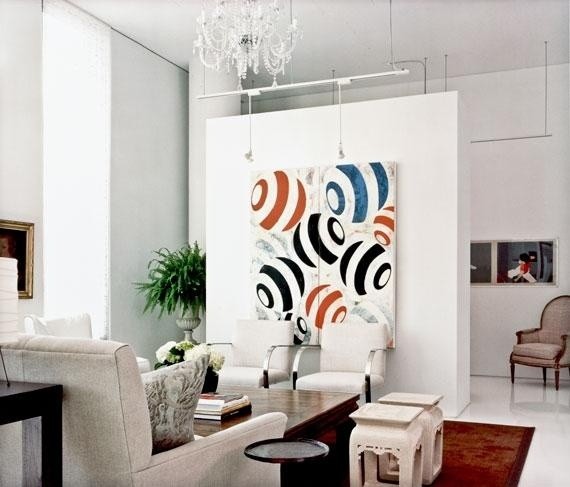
[193,391,253,423]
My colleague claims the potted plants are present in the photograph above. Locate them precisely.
[130,240,206,345]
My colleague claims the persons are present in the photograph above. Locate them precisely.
[508,252,537,282]
[0,234,25,290]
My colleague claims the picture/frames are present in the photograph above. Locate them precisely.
[0,219,35,299]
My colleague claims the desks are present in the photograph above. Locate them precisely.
[0,379,63,487]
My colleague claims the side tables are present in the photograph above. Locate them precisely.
[244,438,331,487]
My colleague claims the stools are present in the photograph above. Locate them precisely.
[347,403,430,487]
[377,392,444,485]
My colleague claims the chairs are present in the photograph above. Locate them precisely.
[511,295,570,391]
[24,315,151,373]
[207,320,295,388]
[291,323,388,402]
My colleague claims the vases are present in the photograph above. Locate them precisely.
[202,366,219,394]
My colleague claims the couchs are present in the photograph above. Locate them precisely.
[0,334,287,487]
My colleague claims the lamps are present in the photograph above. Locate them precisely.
[191,0,303,92]
[336,79,353,158]
[245,93,260,163]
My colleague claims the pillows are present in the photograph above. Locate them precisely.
[141,352,210,455]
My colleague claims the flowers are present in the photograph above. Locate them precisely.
[153,340,225,371]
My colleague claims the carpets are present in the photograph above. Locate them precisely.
[278,415,536,487]
